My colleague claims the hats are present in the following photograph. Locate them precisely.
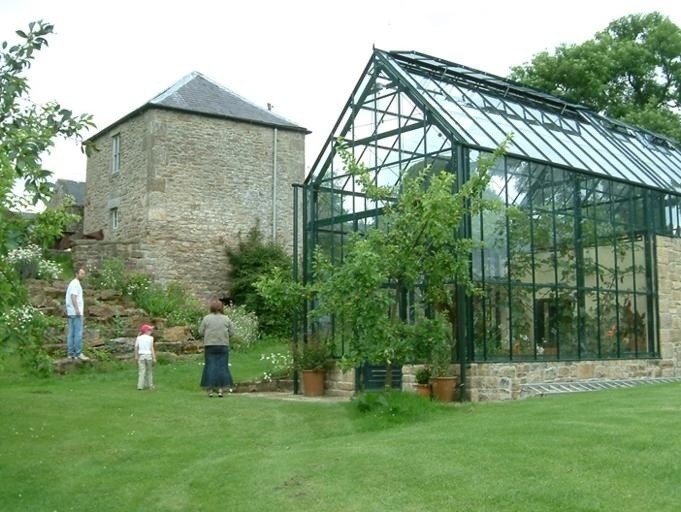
[141,324,154,332]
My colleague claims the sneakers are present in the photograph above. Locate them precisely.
[77,353,89,360]
[148,385,154,390]
[68,354,78,360]
[137,385,146,390]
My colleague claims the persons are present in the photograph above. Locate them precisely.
[64,269,90,361]
[198,300,234,397]
[134,325,157,390]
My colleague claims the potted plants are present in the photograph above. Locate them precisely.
[412,366,431,401]
[429,344,459,402]
[287,329,336,397]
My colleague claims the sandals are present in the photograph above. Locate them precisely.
[209,393,214,397]
[218,392,223,396]
